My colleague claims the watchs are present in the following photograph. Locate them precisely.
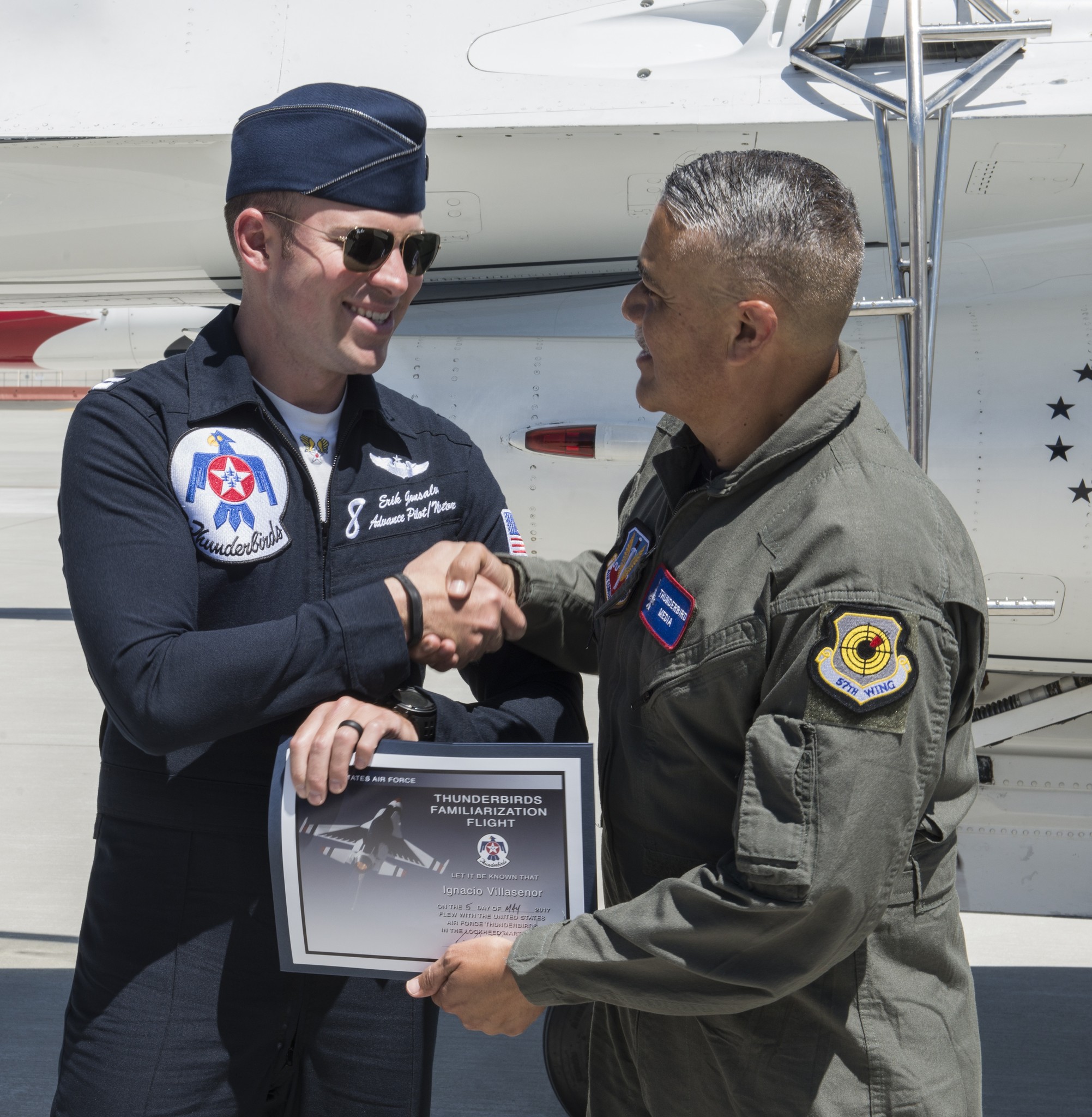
[382,685,437,742]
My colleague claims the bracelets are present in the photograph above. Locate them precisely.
[391,570,426,651]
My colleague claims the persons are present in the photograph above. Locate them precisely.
[50,82,590,1117]
[404,152,989,1117]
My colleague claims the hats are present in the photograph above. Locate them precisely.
[225,83,429,214]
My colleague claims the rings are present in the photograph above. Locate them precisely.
[338,718,366,736]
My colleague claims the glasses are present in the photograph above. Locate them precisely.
[260,210,441,277]
